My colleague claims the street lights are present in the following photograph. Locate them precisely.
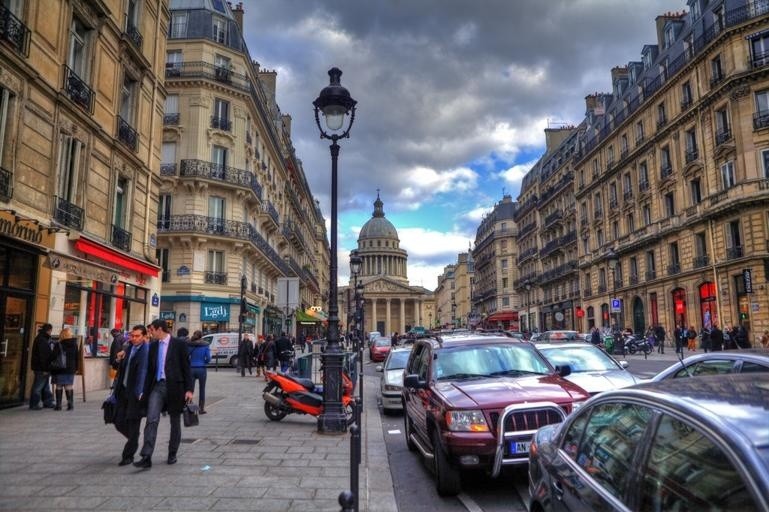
[311,69,359,434]
[349,249,364,374]
[523,279,533,336]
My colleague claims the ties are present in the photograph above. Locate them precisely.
[157,341,164,382]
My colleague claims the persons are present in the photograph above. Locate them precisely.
[391,331,398,351]
[28,323,55,410]
[590,322,752,354]
[236,331,312,377]
[102,324,211,462]
[51,328,79,411]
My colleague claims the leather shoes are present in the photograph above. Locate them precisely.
[133,458,151,468]
[168,451,176,464]
[118,458,133,466]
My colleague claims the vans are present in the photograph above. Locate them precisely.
[202,333,258,368]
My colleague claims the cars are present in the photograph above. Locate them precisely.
[529,340,649,395]
[376,346,415,414]
[649,353,768,382]
[529,372,768,512]
[368,326,591,362]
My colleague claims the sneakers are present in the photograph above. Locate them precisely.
[30,403,54,409]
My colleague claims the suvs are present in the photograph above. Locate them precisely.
[400,332,592,495]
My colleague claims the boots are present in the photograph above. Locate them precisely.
[54,389,63,410]
[65,389,73,410]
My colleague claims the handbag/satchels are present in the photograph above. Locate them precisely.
[258,353,268,362]
[183,398,201,427]
[100,396,118,424]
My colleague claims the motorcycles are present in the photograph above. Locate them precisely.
[624,334,652,355]
[262,367,356,425]
